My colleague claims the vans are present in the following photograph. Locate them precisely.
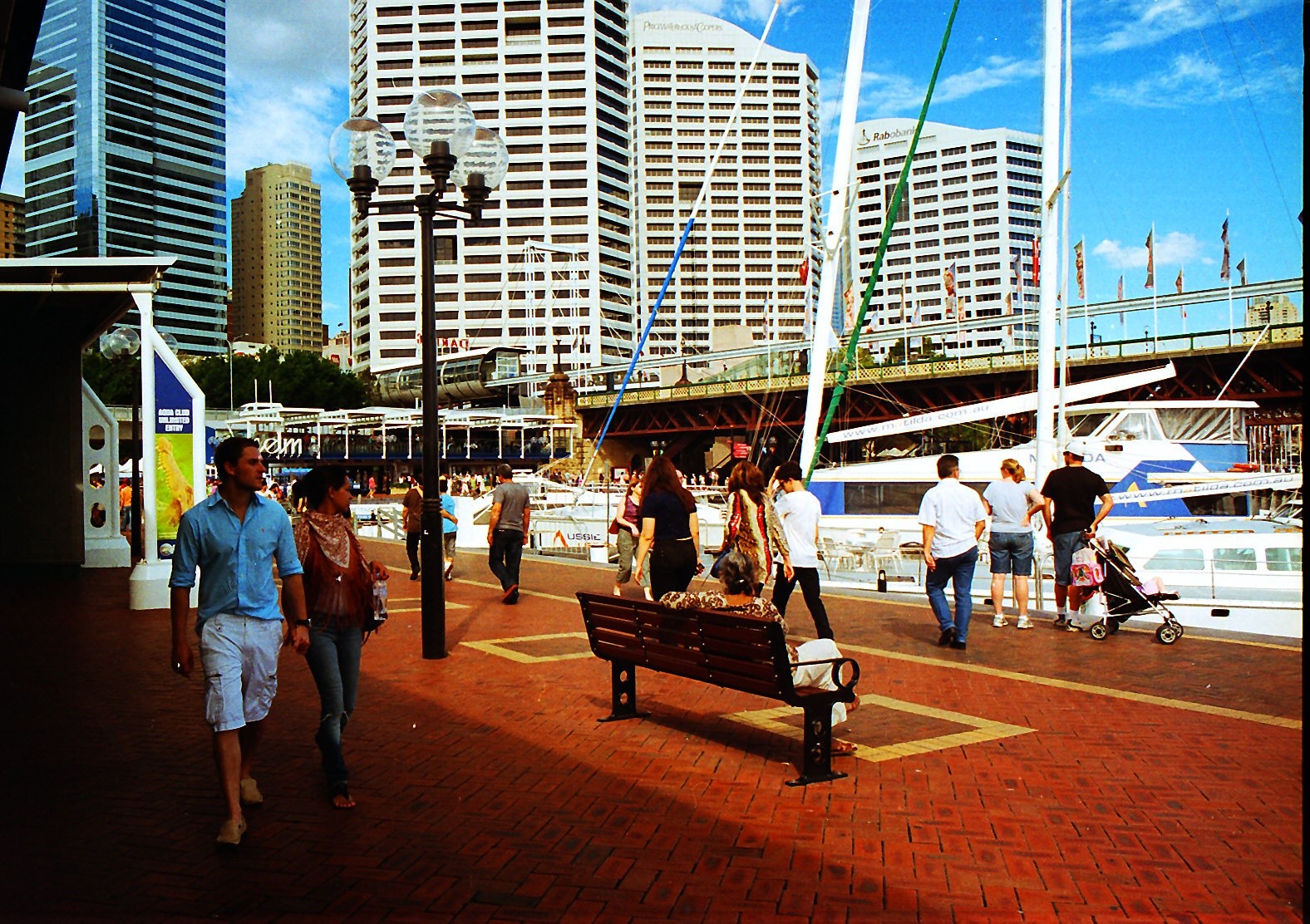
[235,402,282,417]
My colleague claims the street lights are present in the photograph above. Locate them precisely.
[329,89,510,662]
[102,327,181,568]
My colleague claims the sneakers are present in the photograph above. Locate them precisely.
[1017,615,1034,628]
[446,570,452,581]
[993,613,1008,627]
[444,561,454,578]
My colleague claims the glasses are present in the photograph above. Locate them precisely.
[955,468,961,473]
[779,478,789,488]
[1000,467,1005,471]
[634,485,642,489]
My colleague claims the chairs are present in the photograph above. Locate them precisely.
[806,526,905,574]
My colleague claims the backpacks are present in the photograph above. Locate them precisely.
[1069,548,1106,587]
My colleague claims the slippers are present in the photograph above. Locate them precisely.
[327,781,356,810]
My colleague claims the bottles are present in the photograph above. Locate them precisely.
[372,571,387,621]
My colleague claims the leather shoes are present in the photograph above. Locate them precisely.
[216,815,247,844]
[239,779,264,806]
[410,562,421,580]
[509,591,519,605]
[502,584,518,603]
[950,641,966,650]
[938,627,958,645]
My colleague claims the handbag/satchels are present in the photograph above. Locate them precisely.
[608,518,620,534]
[345,526,388,648]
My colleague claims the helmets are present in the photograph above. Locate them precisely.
[1062,439,1087,457]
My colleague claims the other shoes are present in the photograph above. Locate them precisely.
[1066,617,1083,632]
[1052,614,1066,626]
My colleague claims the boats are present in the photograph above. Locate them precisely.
[297,376,1307,627]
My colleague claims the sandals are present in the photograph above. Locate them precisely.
[830,737,858,755]
[844,696,860,714]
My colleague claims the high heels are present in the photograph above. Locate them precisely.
[613,584,620,596]
[643,589,653,602]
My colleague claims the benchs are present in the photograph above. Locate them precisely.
[575,589,860,787]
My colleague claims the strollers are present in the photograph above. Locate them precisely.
[1077,530,1185,644]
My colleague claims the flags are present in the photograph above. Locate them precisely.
[802,212,1251,321]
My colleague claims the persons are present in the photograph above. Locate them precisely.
[983,458,1046,629]
[656,551,862,757]
[436,478,459,578]
[485,463,530,604]
[1040,440,1114,630]
[709,460,796,598]
[167,437,313,843]
[916,454,989,648]
[84,448,741,554]
[633,455,704,601]
[612,478,655,601]
[759,461,838,643]
[287,465,388,809]
[402,473,459,580]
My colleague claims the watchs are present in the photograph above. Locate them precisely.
[294,618,312,628]
